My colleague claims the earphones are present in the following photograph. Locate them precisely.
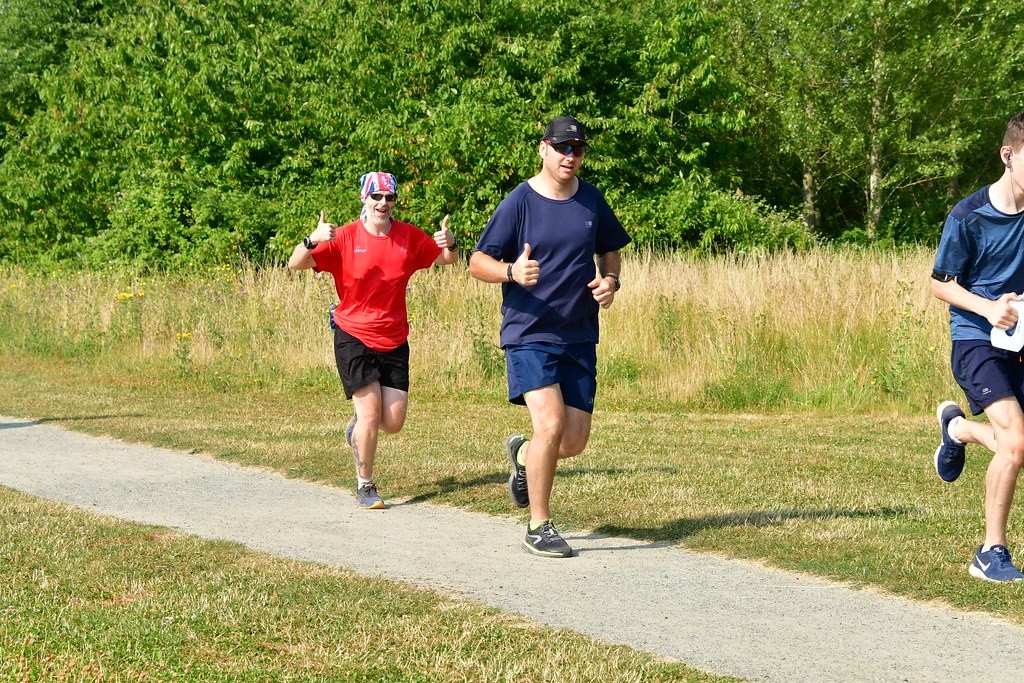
[1006,153,1011,164]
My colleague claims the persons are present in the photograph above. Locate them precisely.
[468,116,633,558]
[930,107,1024,583]
[288,171,460,510]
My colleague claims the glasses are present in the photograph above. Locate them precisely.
[369,193,398,202]
[543,140,585,157]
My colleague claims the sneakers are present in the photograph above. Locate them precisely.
[934,401,970,484]
[344,406,357,446]
[519,518,573,558]
[967,543,1024,585]
[505,433,531,509]
[355,479,385,510]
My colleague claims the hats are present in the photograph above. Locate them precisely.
[540,116,586,144]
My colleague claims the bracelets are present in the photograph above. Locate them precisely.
[448,237,458,251]
[508,264,515,284]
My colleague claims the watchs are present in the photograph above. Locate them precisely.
[603,272,621,291]
[303,236,318,250]
